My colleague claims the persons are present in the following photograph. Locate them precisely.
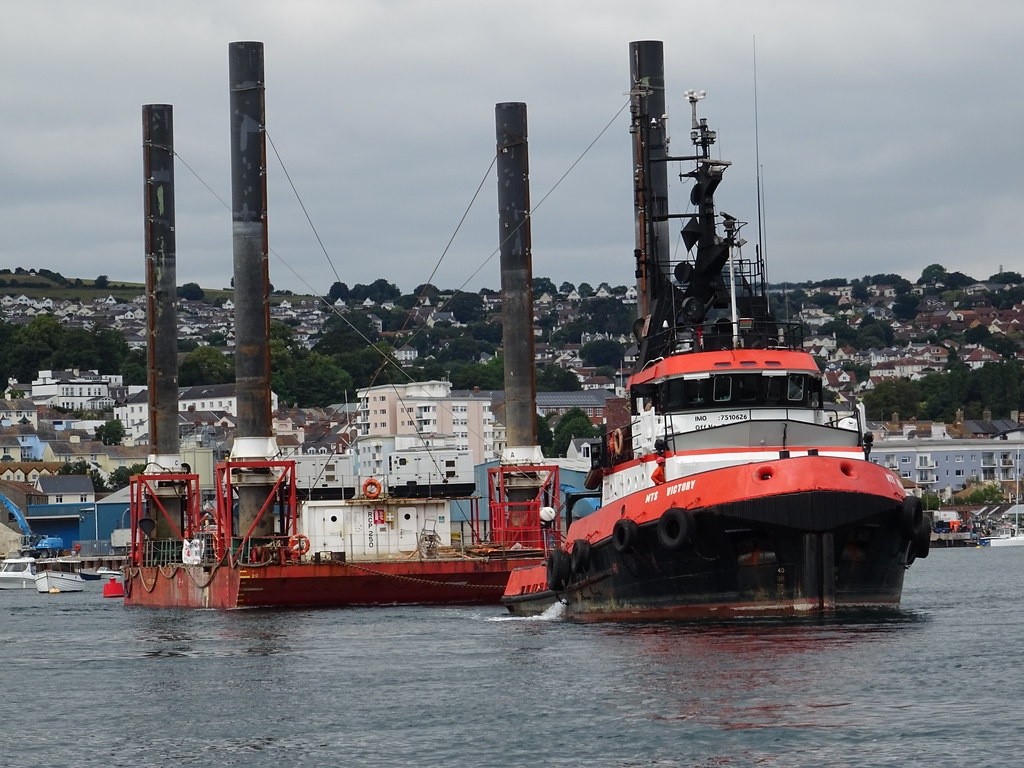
[650,457,668,486]
[931,512,1023,551]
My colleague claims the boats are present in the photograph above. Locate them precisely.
[0,555,38,589]
[34,549,85,592]
[503,88,935,621]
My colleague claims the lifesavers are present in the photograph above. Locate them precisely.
[363,478,382,500]
[288,533,310,556]
[613,427,624,456]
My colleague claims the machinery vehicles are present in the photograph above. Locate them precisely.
[0,492,64,558]
